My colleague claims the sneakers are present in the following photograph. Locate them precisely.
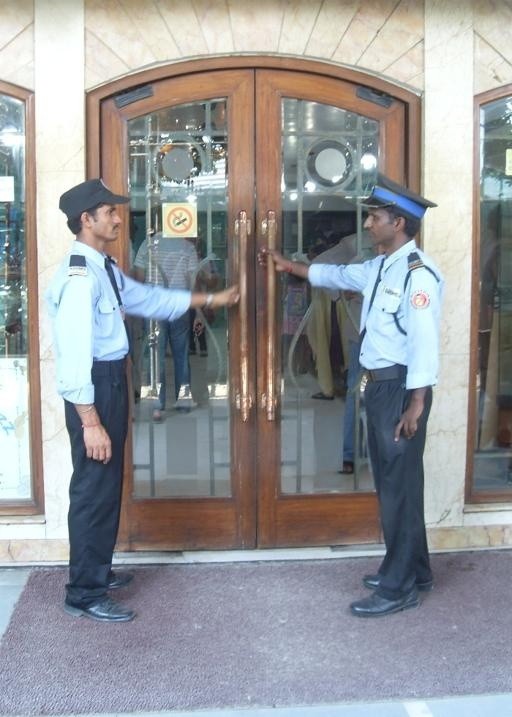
[200,349,208,355]
[188,348,195,354]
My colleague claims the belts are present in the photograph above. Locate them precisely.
[92,358,127,376]
[366,364,400,382]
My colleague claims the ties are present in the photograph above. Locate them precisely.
[104,260,135,366]
[359,259,384,339]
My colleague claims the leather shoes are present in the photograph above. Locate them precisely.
[63,598,137,622]
[362,568,433,591]
[311,391,334,400]
[341,461,353,473]
[109,571,134,590]
[350,581,419,618]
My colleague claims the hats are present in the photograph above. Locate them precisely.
[58,178,131,221]
[357,173,438,221]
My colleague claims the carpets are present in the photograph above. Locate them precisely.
[0,546,512,716]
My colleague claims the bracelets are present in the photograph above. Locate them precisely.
[80,421,102,428]
[75,404,98,414]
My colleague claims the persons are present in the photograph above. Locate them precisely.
[125,203,222,423]
[46,174,243,624]
[256,169,446,619]
[282,211,385,476]
